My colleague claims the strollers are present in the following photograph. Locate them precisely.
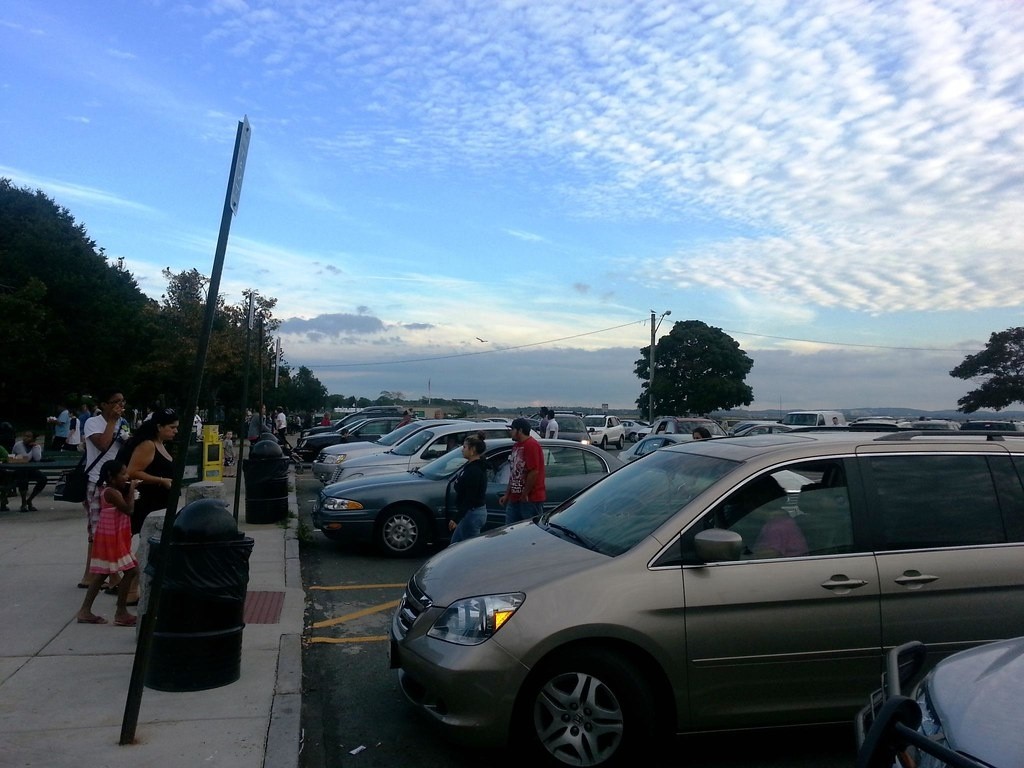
[274,433,304,475]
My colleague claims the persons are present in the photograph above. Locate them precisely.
[435,408,444,419]
[127,408,181,606]
[448,431,487,542]
[740,475,808,560]
[446,436,459,453]
[499,418,546,524]
[545,410,558,438]
[340,429,349,443]
[76,460,138,626]
[77,391,130,596]
[395,410,411,429]
[322,412,333,426]
[0,404,315,512]
[694,428,712,441]
[540,407,548,439]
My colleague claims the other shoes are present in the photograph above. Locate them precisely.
[222,473,237,478]
[0,506,10,511]
[116,597,140,606]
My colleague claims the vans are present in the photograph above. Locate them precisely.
[782,411,846,431]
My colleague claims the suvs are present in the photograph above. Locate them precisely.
[581,414,626,450]
[849,415,1024,437]
[325,422,557,487]
[384,429,1024,767]
[650,416,727,437]
[540,411,589,444]
[300,406,414,440]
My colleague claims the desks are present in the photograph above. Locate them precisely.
[0,460,87,469]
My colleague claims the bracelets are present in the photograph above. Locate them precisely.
[159,476,162,486]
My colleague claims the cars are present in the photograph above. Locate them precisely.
[851,634,1024,768]
[729,421,794,437]
[296,416,408,461]
[483,418,540,431]
[616,433,731,467]
[618,420,657,442]
[311,418,482,481]
[312,438,630,560]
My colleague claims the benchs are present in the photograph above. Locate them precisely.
[23,480,66,485]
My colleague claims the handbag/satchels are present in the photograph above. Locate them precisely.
[54,467,90,503]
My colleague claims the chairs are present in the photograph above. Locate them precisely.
[792,483,852,551]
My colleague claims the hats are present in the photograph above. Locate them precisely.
[506,418,532,432]
[152,408,176,417]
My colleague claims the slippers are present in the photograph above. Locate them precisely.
[19,509,29,512]
[113,615,137,627]
[104,583,121,595]
[27,507,37,511]
[77,616,108,624]
[78,581,109,590]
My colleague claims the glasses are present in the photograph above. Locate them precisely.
[107,399,127,406]
